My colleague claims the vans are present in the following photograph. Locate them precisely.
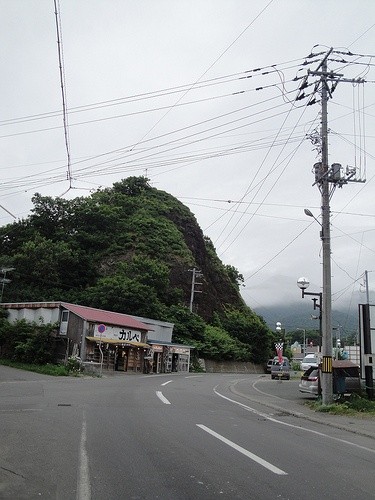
[300,353,319,370]
[267,359,274,372]
[272,356,290,380]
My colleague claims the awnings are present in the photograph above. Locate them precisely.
[85,336,152,349]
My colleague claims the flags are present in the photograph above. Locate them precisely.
[274,342,284,363]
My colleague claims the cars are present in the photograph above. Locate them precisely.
[299,366,361,396]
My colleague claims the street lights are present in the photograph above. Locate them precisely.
[297,278,333,406]
[304,209,323,229]
[275,322,306,352]
